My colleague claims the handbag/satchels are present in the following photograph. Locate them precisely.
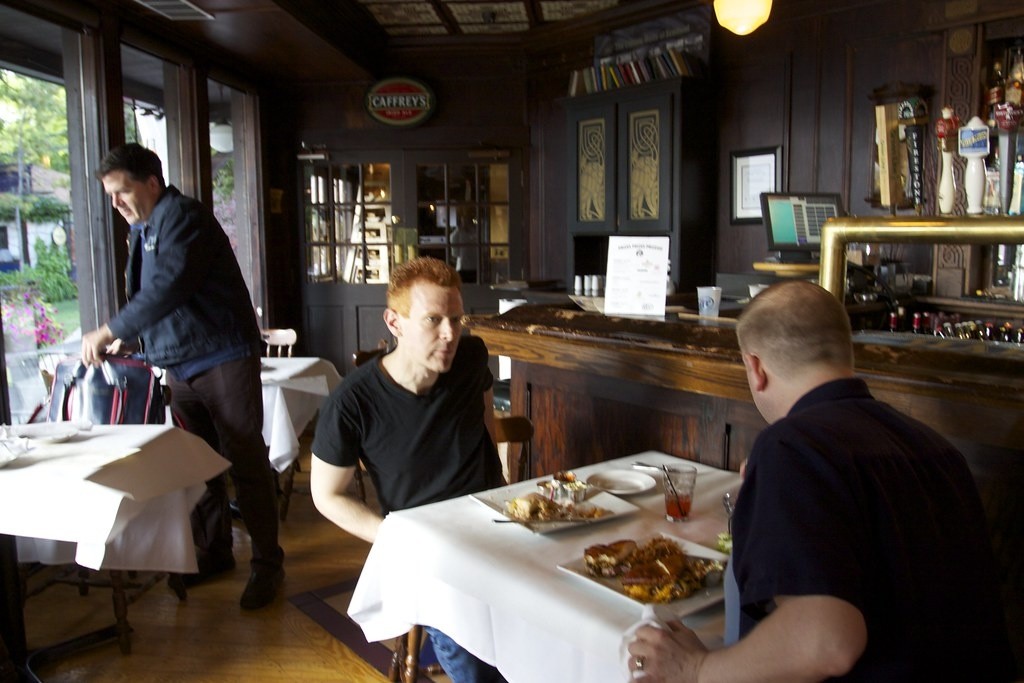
[26,353,191,440]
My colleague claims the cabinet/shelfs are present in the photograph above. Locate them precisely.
[559,76,720,294]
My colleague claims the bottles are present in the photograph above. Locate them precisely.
[889,306,1024,344]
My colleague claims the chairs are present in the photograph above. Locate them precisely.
[280,335,389,525]
[389,416,533,683]
[77,385,173,594]
[257,329,304,473]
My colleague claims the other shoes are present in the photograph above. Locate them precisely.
[240,562,285,608]
[166,549,236,588]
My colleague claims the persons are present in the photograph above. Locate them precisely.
[311,257,503,683]
[81,143,286,613]
[628,281,1024,683]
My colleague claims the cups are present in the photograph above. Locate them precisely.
[574,275,606,295]
[697,286,722,318]
[660,463,698,523]
[721,488,742,537]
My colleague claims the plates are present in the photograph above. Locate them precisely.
[36,428,81,442]
[468,477,640,535]
[556,530,728,619]
[585,469,657,495]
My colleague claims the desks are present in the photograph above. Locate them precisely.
[158,353,345,522]
[345,449,745,683]
[0,422,233,655]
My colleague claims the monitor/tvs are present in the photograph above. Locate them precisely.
[759,193,847,265]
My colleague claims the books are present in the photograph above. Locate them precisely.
[568,47,694,99]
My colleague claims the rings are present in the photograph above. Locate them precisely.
[635,657,643,670]
